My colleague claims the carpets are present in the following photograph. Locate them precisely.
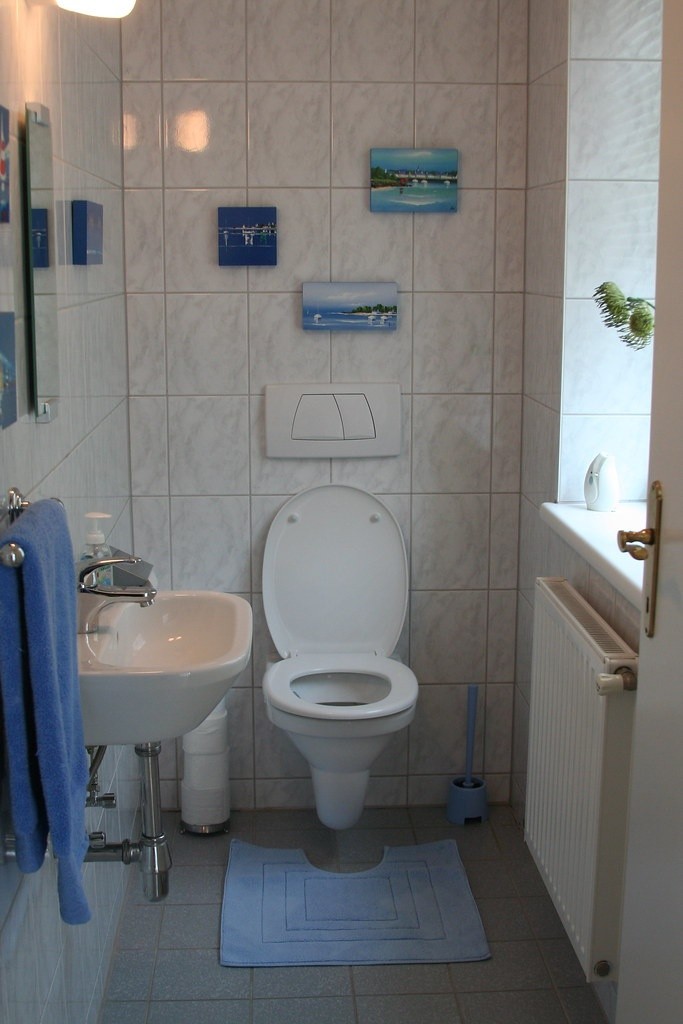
[219,838,492,968]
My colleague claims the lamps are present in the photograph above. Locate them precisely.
[55,0,139,19]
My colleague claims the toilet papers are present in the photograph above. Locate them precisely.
[177,696,232,828]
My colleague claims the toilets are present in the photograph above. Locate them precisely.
[257,481,419,834]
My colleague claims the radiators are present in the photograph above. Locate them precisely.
[523,577,638,984]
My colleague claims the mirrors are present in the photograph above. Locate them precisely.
[26,102,58,420]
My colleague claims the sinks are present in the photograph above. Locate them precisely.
[65,585,255,750]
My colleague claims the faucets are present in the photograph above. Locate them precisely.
[69,556,160,636]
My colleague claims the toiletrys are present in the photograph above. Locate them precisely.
[79,510,116,593]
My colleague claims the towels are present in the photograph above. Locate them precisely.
[1,497,95,927]
